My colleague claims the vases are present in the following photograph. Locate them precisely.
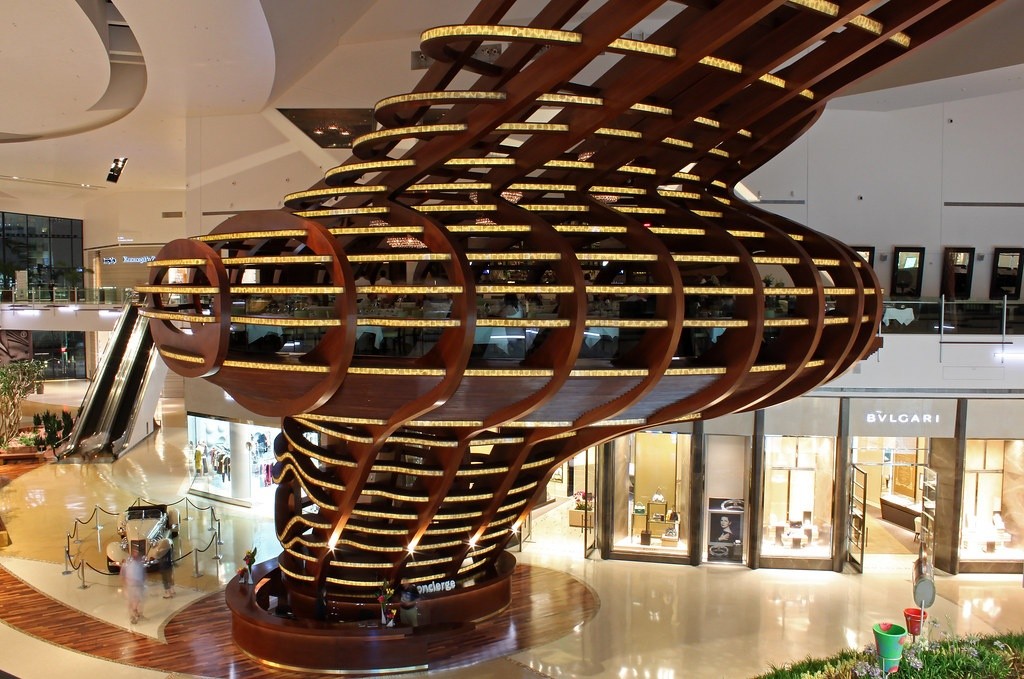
[247,569,253,584]
[569,510,594,527]
[239,578,244,583]
[7,446,38,454]
[386,619,394,627]
[382,609,386,623]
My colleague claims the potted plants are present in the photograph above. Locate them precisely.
[36,381,44,394]
[762,273,785,318]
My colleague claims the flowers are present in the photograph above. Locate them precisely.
[373,578,397,618]
[574,491,593,510]
[8,428,49,447]
[237,546,257,576]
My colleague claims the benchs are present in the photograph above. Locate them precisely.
[0,452,44,465]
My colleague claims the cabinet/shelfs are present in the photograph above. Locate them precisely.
[631,501,675,543]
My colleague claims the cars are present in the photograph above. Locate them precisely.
[106,504,181,575]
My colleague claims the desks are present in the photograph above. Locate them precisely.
[883,305,916,329]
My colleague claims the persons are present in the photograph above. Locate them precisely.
[160,540,176,598]
[714,515,736,560]
[120,546,146,624]
[48,281,55,301]
[308,271,735,357]
